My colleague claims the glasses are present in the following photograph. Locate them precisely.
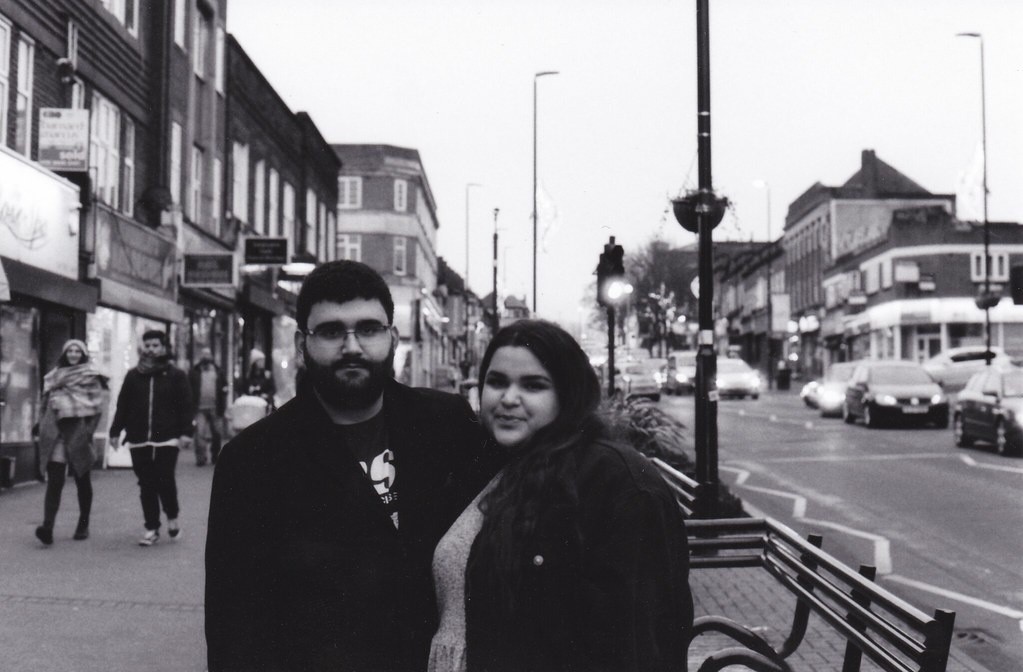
[301,317,391,345]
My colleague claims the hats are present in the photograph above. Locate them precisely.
[62,339,89,358]
[249,349,266,365]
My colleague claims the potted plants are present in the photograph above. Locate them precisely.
[673,190,732,234]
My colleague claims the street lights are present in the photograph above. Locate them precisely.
[533,69,559,314]
[464,183,480,363]
[958,32,1000,366]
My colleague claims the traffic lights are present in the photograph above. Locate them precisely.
[601,243,625,306]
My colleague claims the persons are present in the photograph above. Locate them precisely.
[111,329,197,546]
[188,348,226,467]
[429,319,694,672]
[205,259,487,672]
[35,337,109,543]
[459,354,470,380]
[237,347,277,417]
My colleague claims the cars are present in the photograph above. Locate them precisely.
[841,362,949,427]
[922,346,1013,394]
[801,363,862,417]
[951,364,1023,456]
[589,346,762,402]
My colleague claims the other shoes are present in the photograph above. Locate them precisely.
[71,521,88,539]
[167,512,180,538]
[139,527,160,544]
[211,451,222,464]
[196,452,207,466]
[35,527,52,543]
